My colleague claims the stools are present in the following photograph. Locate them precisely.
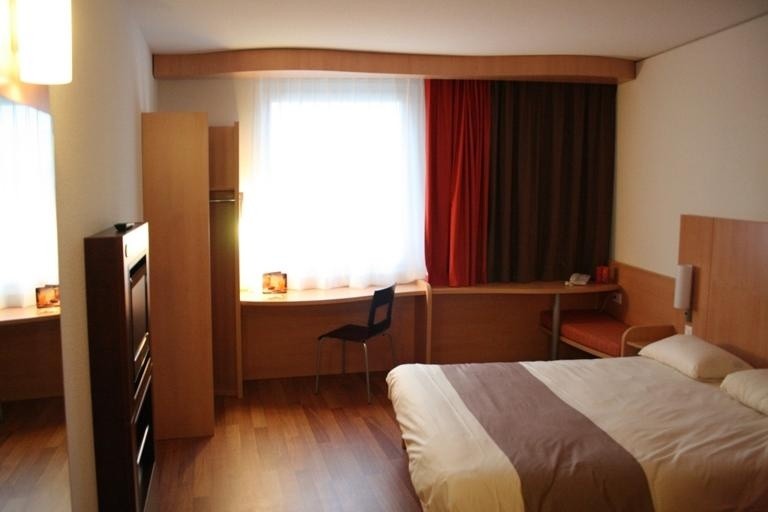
[535,305,634,361]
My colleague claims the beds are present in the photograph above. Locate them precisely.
[383,354,767,512]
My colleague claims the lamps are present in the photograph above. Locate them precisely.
[670,264,693,326]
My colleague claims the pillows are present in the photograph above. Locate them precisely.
[639,334,768,417]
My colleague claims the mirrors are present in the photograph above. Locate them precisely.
[0,93,74,512]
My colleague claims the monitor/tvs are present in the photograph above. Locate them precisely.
[130,264,151,384]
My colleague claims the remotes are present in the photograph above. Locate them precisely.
[113,222,135,231]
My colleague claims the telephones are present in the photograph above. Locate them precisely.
[568,273,590,285]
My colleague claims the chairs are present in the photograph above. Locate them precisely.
[314,283,399,404]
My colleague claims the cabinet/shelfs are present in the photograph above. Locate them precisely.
[142,111,243,441]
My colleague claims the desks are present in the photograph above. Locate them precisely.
[239,278,621,383]
